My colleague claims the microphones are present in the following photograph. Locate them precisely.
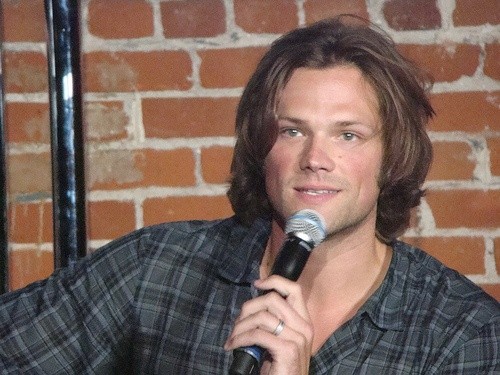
[226,208,328,375]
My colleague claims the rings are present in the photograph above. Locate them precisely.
[271,320,287,336]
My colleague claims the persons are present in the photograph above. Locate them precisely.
[3,15,499,375]
